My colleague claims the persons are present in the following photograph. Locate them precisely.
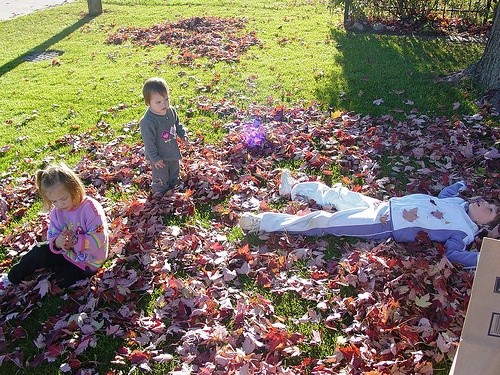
[240,171,500,267]
[8,167,108,287]
[140,78,190,197]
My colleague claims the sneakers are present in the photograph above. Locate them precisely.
[1,273,13,301]
[279,168,298,195]
[239,211,261,233]
